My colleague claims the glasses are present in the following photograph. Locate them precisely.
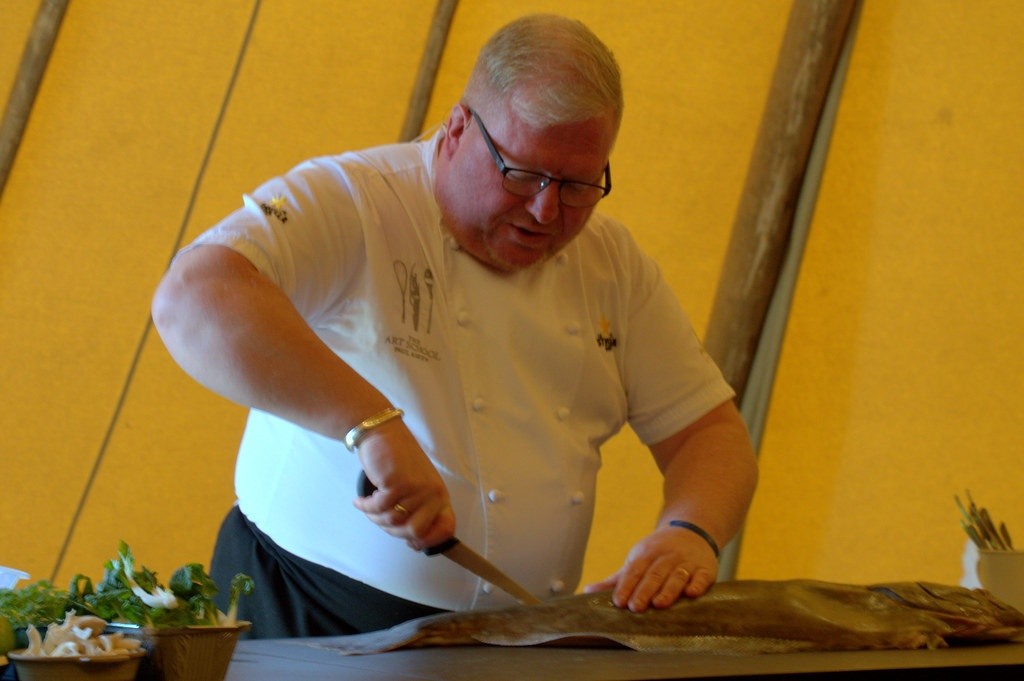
[468,105,612,209]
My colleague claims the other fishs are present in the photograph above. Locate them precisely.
[295,581,1023,654]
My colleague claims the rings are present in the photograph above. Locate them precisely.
[676,567,688,575]
[395,504,408,515]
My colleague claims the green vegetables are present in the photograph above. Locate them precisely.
[0,538,256,635]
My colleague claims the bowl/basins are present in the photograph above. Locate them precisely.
[104,620,252,680]
[7,646,148,681]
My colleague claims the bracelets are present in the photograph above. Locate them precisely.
[665,521,719,558]
[343,407,404,453]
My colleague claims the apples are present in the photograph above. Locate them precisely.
[0,614,16,656]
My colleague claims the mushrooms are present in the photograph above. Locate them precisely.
[22,608,144,657]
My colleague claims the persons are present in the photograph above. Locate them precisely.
[151,14,758,641]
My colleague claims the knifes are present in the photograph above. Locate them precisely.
[357,470,538,606]
